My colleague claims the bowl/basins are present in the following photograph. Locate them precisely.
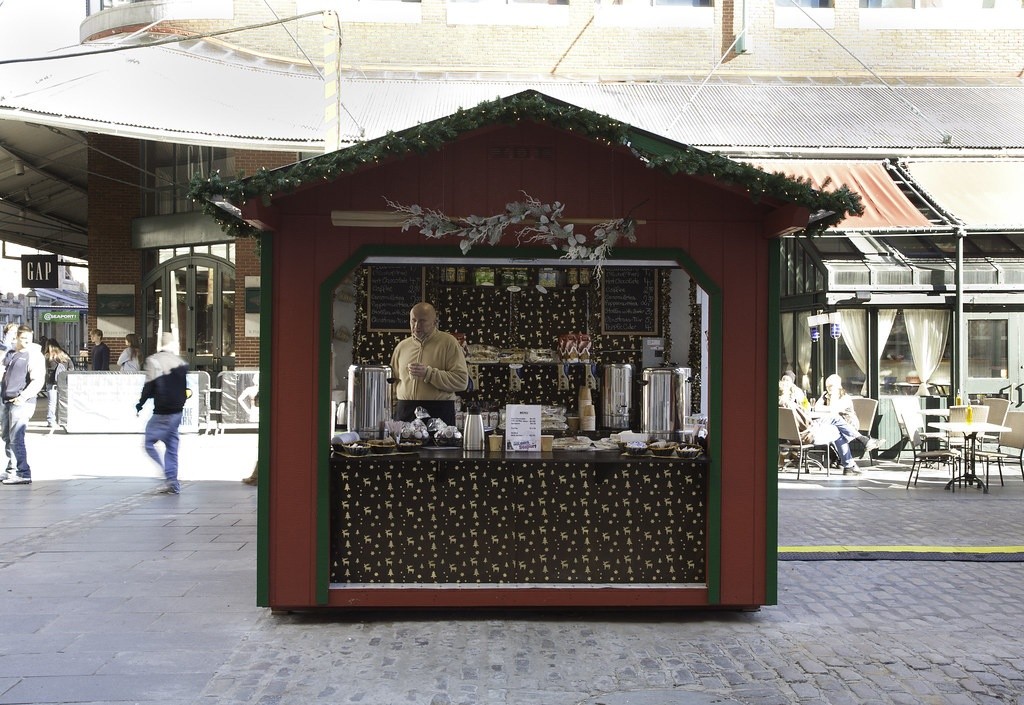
[396,430,462,451]
[623,441,703,458]
[342,442,371,455]
[367,440,396,453]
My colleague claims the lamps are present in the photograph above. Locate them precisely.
[14,161,25,176]
[25,190,32,201]
[855,291,871,302]
[19,211,25,220]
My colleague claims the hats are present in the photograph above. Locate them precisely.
[826,374,842,387]
[781,370,796,383]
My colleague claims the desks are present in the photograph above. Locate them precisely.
[919,408,958,469]
[781,411,836,473]
[928,421,1013,495]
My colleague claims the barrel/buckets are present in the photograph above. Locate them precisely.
[344,364,396,439]
[597,363,638,427]
[637,365,694,442]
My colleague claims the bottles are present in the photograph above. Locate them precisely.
[427,265,591,288]
[965,402,972,425]
[801,390,808,410]
[956,393,961,405]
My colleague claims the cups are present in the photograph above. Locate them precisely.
[489,435,503,452]
[568,386,595,431]
[809,398,816,412]
[541,435,554,451]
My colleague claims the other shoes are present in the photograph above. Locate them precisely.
[863,435,886,452]
[830,460,844,469]
[843,464,868,476]
[243,476,257,485]
[154,485,179,496]
[0,472,32,484]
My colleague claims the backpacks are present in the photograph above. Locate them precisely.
[52,358,67,383]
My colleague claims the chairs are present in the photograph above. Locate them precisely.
[778,397,1024,493]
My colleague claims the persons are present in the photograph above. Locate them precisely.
[90,328,110,370]
[242,392,259,486]
[390,303,468,446]
[238,372,259,423]
[136,332,188,494]
[117,334,145,372]
[0,322,46,484]
[779,370,886,476]
[39,335,74,427]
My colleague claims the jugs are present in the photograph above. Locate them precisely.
[336,400,347,427]
[463,404,485,451]
